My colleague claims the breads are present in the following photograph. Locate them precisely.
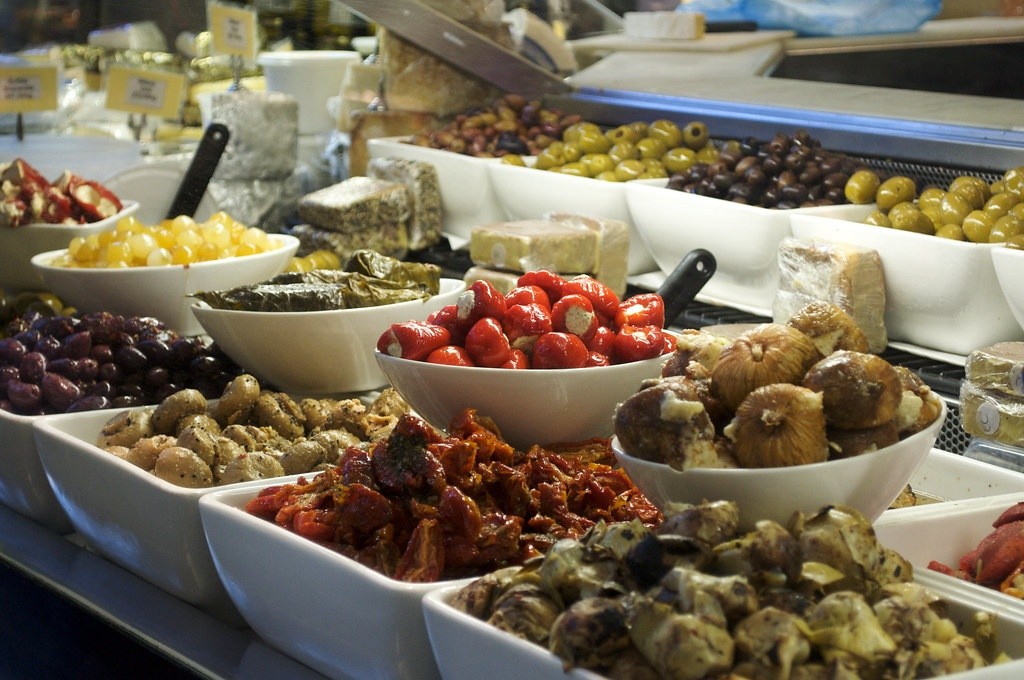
[773,238,888,353]
[623,10,705,39]
[203,0,521,260]
[0,19,296,149]
[460,209,629,299]
[958,340,1024,448]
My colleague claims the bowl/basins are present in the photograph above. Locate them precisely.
[1,51,1024,680]
